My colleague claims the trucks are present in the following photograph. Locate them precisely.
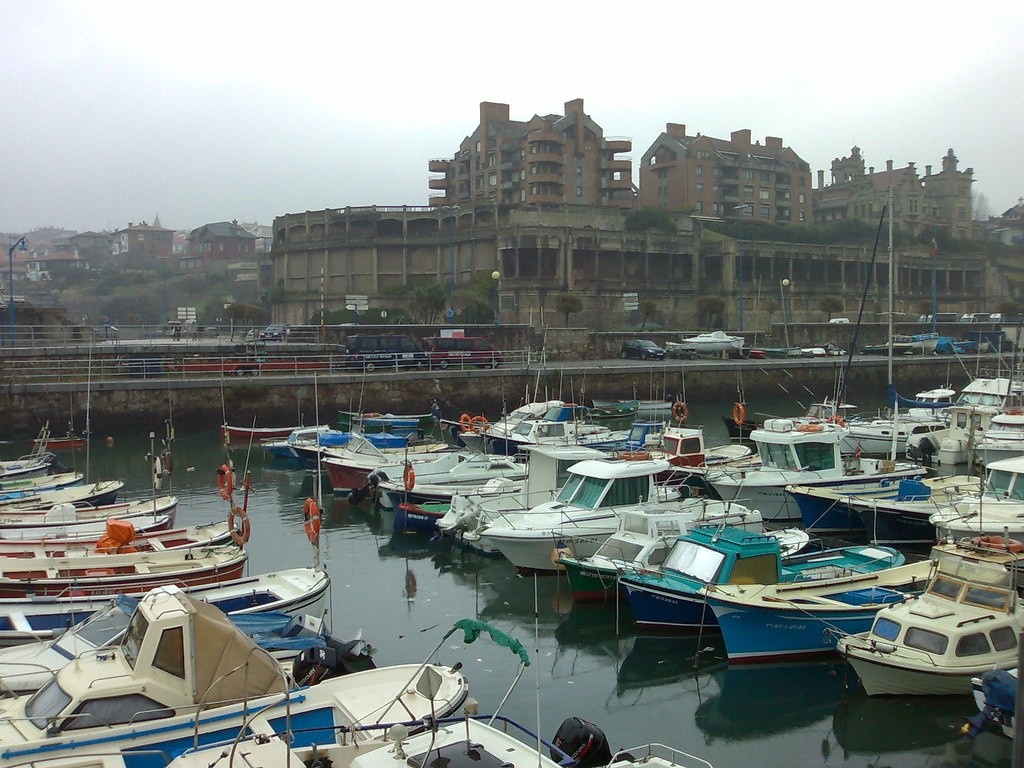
[917,312,958,323]
[989,312,1022,322]
[960,312,990,322]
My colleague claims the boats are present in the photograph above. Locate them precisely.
[665,330,745,360]
[0,184,1024,767]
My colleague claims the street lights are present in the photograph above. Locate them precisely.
[258,236,274,326]
[491,269,501,327]
[782,278,790,323]
[442,203,461,324]
[6,234,29,349]
[155,256,172,326]
[732,205,749,330]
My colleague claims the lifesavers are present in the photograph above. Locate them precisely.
[227,506,251,546]
[304,497,320,543]
[620,451,651,460]
[403,464,416,490]
[672,401,689,424]
[800,424,824,431]
[217,463,233,500]
[973,532,1024,554]
[732,402,746,424]
[560,404,577,408]
[151,448,172,492]
[459,413,490,435]
[827,416,845,429]
[1007,409,1023,415]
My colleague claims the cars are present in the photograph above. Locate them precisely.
[620,339,667,361]
[829,318,849,324]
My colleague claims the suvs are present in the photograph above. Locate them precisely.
[345,333,429,373]
[422,337,507,370]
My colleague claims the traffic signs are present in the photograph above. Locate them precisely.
[345,295,368,300]
[344,299,368,305]
[345,304,368,311]
